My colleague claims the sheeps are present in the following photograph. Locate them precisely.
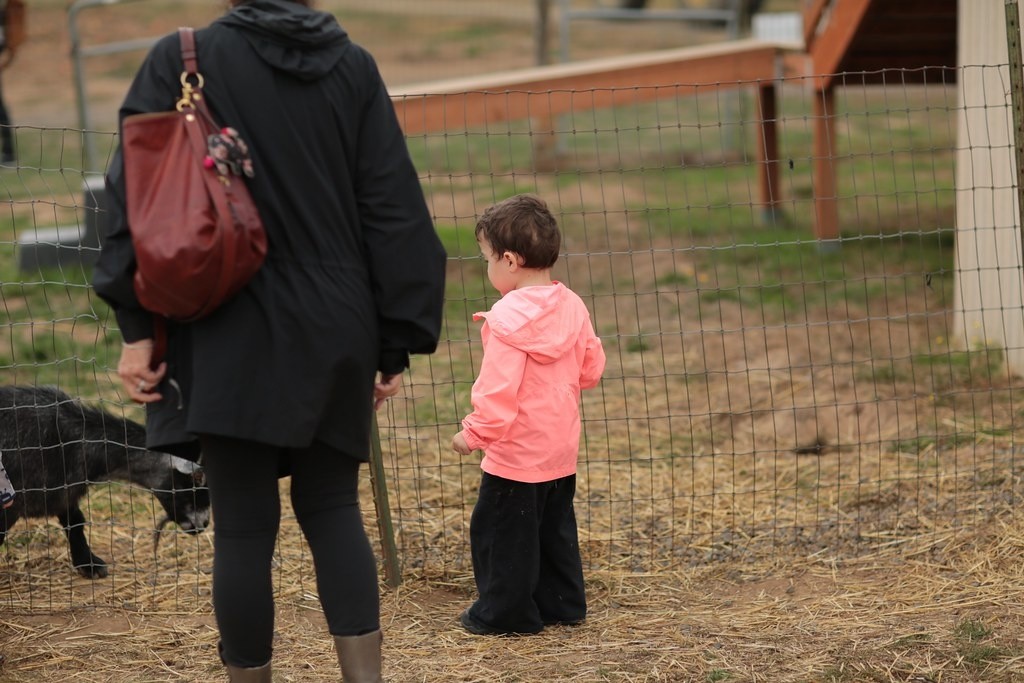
[0,383,213,578]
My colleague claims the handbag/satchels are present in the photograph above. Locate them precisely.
[122,26,268,321]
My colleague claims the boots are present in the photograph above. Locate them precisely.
[330,625,382,683]
[215,640,272,683]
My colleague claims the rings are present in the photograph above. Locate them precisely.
[137,380,146,389]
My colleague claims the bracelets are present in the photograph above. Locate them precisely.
[122,342,153,350]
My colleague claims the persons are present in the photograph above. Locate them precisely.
[94,1,447,683]
[453,197,612,634]
[0,0,25,164]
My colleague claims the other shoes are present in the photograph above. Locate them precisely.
[449,607,492,636]
